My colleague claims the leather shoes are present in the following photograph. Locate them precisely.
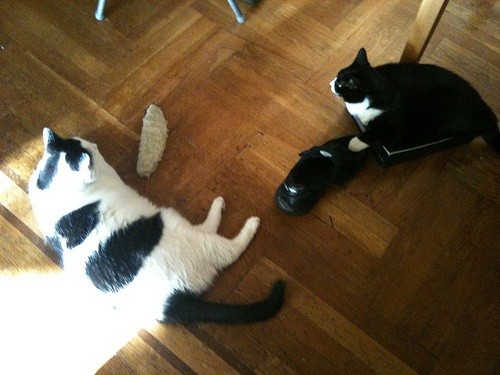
[273,135,358,217]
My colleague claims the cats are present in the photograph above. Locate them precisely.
[28,124,287,326]
[329,47,500,159]
